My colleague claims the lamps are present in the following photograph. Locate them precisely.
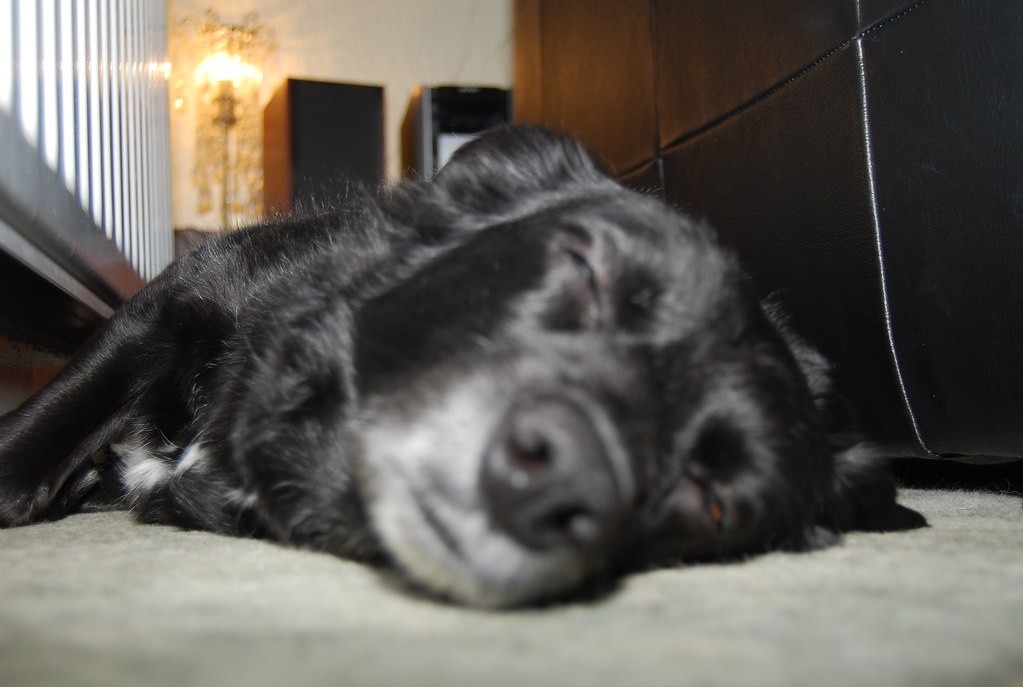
[173,6,277,233]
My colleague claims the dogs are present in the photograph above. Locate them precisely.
[0,121,900,615]
